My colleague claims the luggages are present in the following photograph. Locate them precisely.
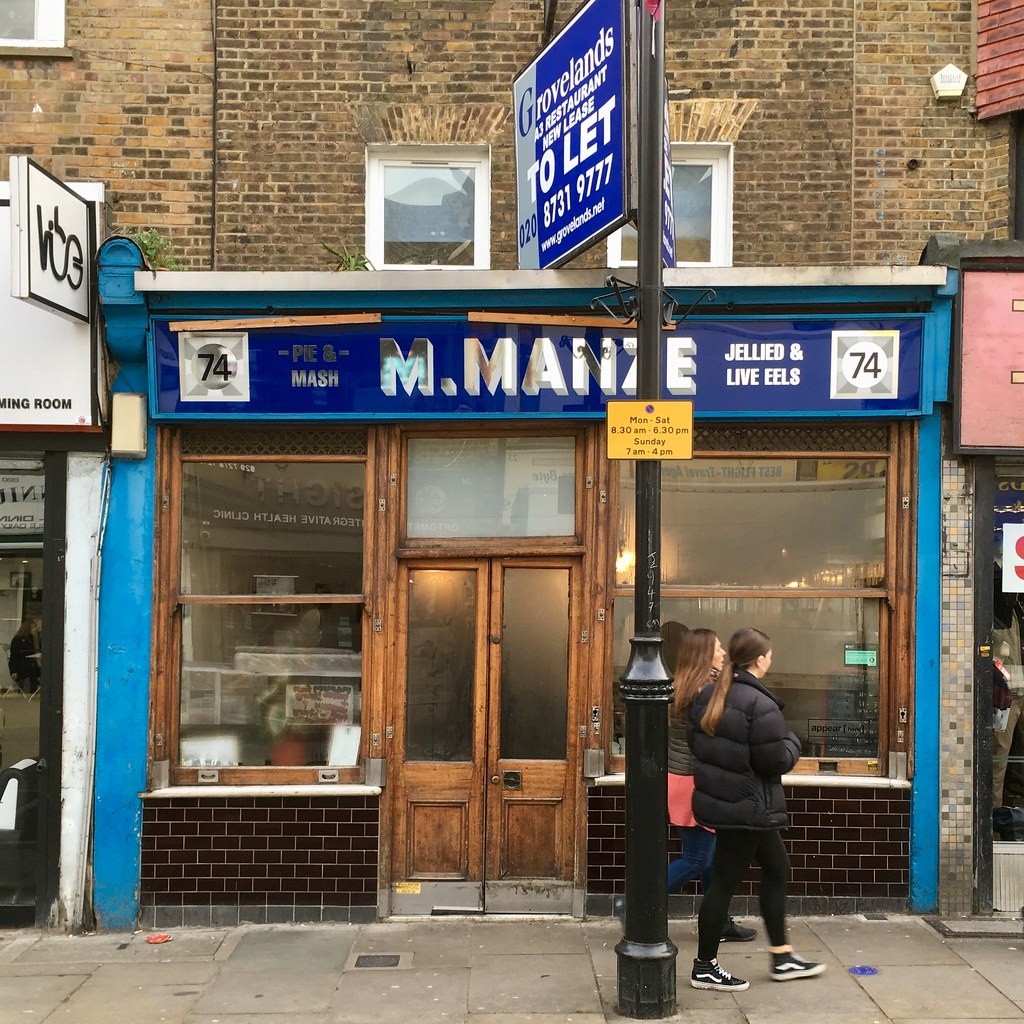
[993,806,1024,842]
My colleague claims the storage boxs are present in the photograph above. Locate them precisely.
[181,646,361,725]
[180,735,240,767]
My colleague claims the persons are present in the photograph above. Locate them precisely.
[684,627,830,992]
[8,620,43,695]
[614,626,760,943]
[988,535,1024,817]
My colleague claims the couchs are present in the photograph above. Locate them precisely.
[0,757,36,904]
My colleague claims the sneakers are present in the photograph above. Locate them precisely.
[720,915,757,943]
[616,898,624,933]
[769,951,827,980]
[690,958,750,992]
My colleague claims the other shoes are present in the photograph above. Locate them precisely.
[30,690,39,694]
[18,687,27,695]
